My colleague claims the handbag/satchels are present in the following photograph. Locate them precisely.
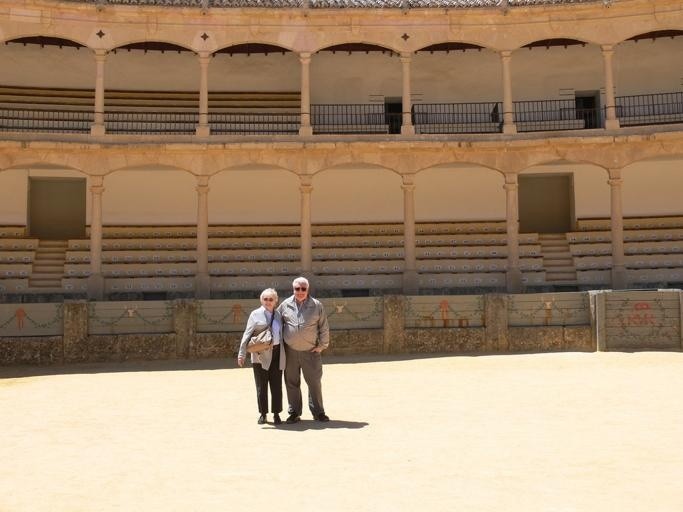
[246,308,274,353]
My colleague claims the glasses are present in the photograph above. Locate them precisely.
[295,287,306,291]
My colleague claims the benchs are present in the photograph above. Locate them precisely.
[311,112,680,135]
[1,223,39,296]
[566,214,682,289]
[3,85,304,134]
[61,220,550,294]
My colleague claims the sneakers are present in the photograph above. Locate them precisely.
[258,415,267,424]
[286,414,300,424]
[274,415,282,425]
[314,415,329,422]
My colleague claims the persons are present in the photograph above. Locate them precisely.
[277,277,330,423]
[238,288,287,424]
